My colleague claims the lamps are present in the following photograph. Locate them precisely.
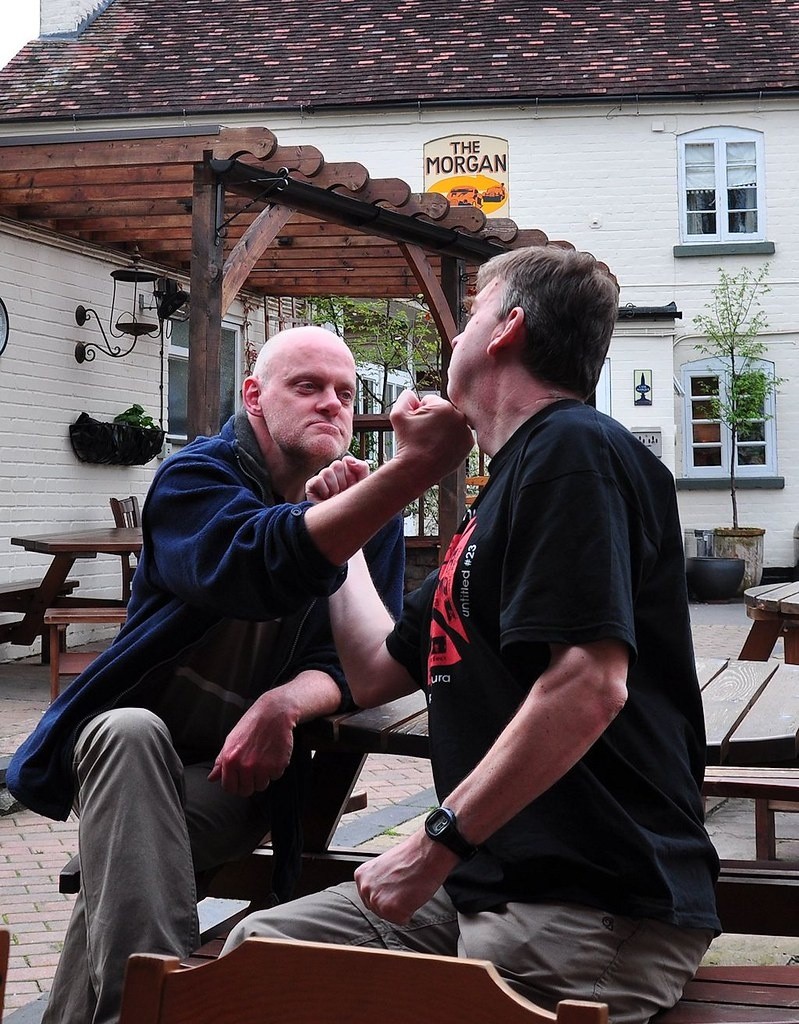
[138,292,193,322]
[75,245,160,364]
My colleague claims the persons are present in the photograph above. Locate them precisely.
[219,246,723,1024]
[5,325,474,1024]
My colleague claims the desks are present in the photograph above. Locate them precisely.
[247,654,798,945]
[12,527,146,646]
[737,582,799,664]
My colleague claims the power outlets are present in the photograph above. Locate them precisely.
[158,443,173,459]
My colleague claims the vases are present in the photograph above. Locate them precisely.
[687,555,745,604]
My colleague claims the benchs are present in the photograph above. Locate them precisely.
[59,767,799,1024]
[0,575,130,702]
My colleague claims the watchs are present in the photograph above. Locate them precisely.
[425,808,477,862]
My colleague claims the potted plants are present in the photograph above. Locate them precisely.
[70,404,165,466]
[692,258,790,595]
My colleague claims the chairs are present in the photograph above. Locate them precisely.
[110,496,144,630]
